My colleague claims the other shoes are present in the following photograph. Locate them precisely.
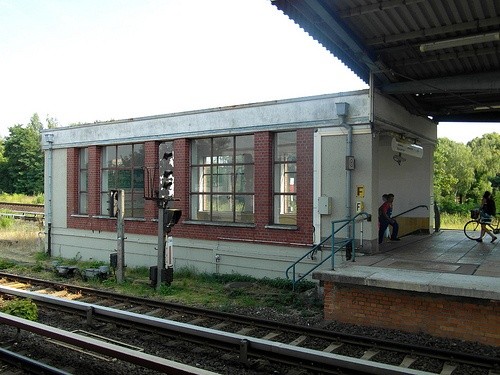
[476,238,482,242]
[491,237,497,243]
[391,237,401,241]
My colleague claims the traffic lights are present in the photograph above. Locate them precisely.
[158,144,177,198]
[162,209,182,233]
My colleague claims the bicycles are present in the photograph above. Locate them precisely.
[463,209,500,241]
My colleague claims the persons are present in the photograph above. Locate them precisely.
[378,194,401,244]
[476,191,497,243]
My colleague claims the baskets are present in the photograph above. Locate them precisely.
[471,210,480,219]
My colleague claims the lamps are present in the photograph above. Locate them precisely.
[419,32,500,52]
